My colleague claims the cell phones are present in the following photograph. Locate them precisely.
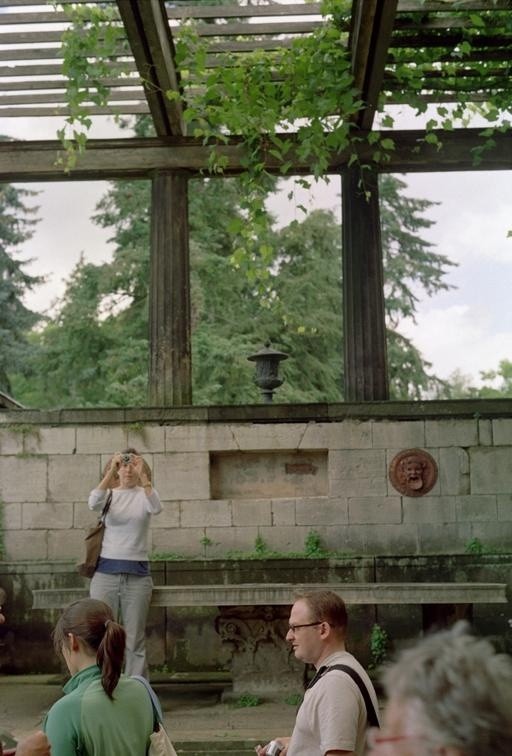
[265,740,285,756]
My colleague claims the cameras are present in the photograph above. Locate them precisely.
[120,454,132,463]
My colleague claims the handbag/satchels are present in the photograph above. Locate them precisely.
[76,487,113,578]
[130,677,178,756]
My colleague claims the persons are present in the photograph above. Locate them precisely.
[253,591,379,756]
[89,449,165,677]
[374,622,512,755]
[44,597,163,755]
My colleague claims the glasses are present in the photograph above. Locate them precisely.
[287,622,333,632]
[365,727,444,751]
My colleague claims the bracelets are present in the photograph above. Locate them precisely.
[143,481,153,488]
